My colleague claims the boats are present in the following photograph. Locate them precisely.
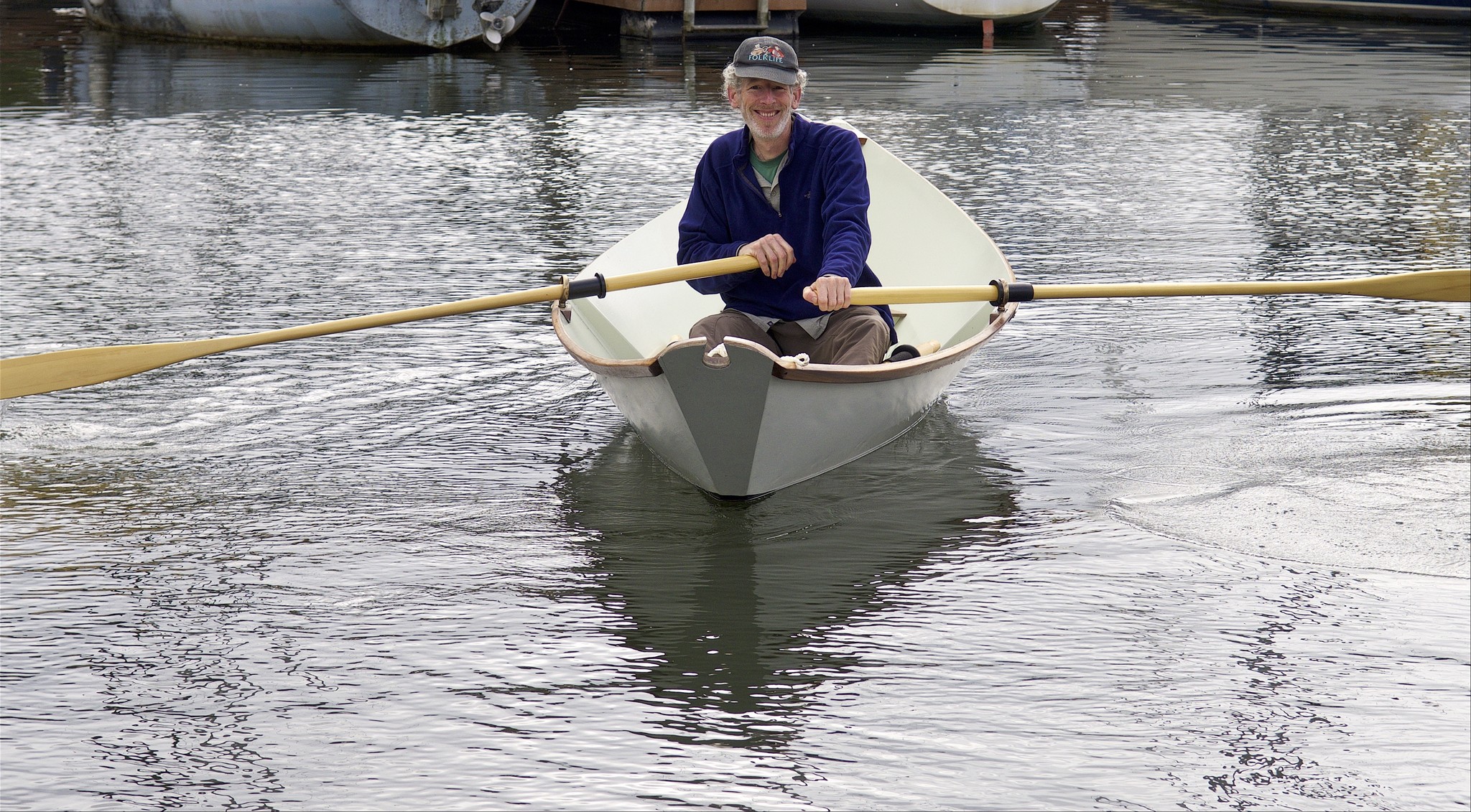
[548,117,1020,510]
[802,0,1062,34]
[46,1,539,61]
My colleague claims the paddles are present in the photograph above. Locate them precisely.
[2,254,760,402]
[810,266,1471,307]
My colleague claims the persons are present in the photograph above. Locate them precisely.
[677,36,899,364]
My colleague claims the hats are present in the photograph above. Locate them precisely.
[733,37,798,85]
